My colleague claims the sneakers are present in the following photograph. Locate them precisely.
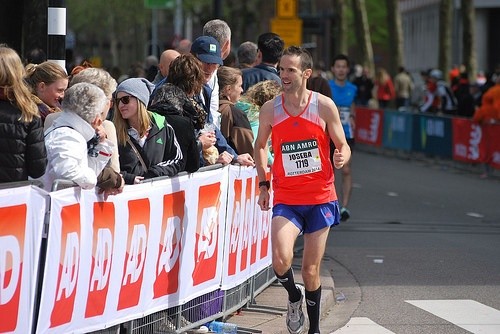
[286,286,305,334]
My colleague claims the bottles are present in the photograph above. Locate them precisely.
[205,321,237,334]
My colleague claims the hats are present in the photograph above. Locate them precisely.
[430,69,443,79]
[115,78,155,108]
[191,36,223,65]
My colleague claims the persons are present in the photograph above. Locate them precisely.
[0,19,286,201]
[254,44,354,334]
[325,54,358,223]
[307,58,500,124]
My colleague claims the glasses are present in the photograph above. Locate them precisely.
[112,96,130,106]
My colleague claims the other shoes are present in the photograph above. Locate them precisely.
[340,208,350,221]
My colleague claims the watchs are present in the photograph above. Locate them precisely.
[258,180,270,190]
